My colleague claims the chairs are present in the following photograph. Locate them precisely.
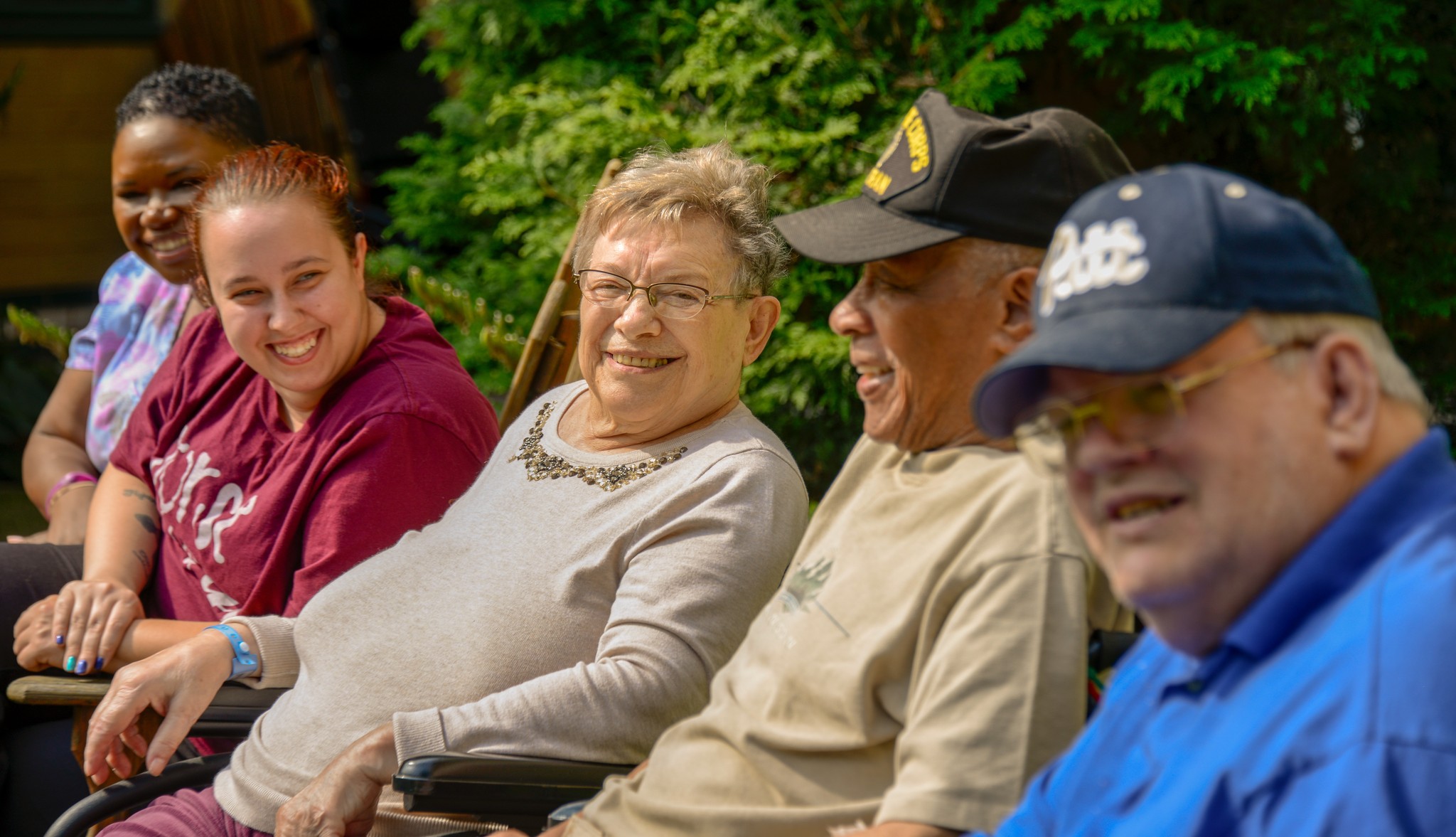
[7,157,627,837]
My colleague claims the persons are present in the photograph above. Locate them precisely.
[496,83,1140,837]
[86,141,806,837]
[951,162,1456,836]
[6,61,270,534]
[0,142,503,837]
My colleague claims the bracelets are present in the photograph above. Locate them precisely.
[41,471,95,514]
[204,624,257,681]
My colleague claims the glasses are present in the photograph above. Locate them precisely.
[1010,334,1319,478]
[572,270,758,319]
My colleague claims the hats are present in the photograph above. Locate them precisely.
[970,165,1382,436]
[772,86,1138,263]
